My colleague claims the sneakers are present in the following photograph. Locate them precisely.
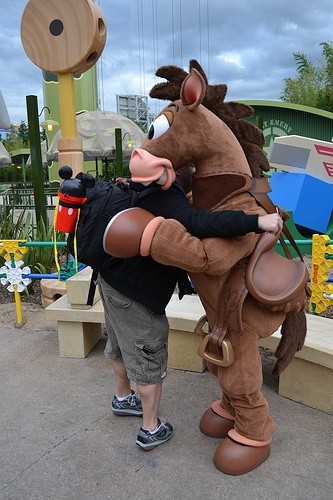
[136,418,174,451]
[111,389,142,416]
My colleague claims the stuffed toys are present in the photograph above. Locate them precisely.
[102,60,311,476]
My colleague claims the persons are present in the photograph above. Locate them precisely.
[93,165,283,451]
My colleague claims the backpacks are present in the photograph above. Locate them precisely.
[66,183,186,268]
